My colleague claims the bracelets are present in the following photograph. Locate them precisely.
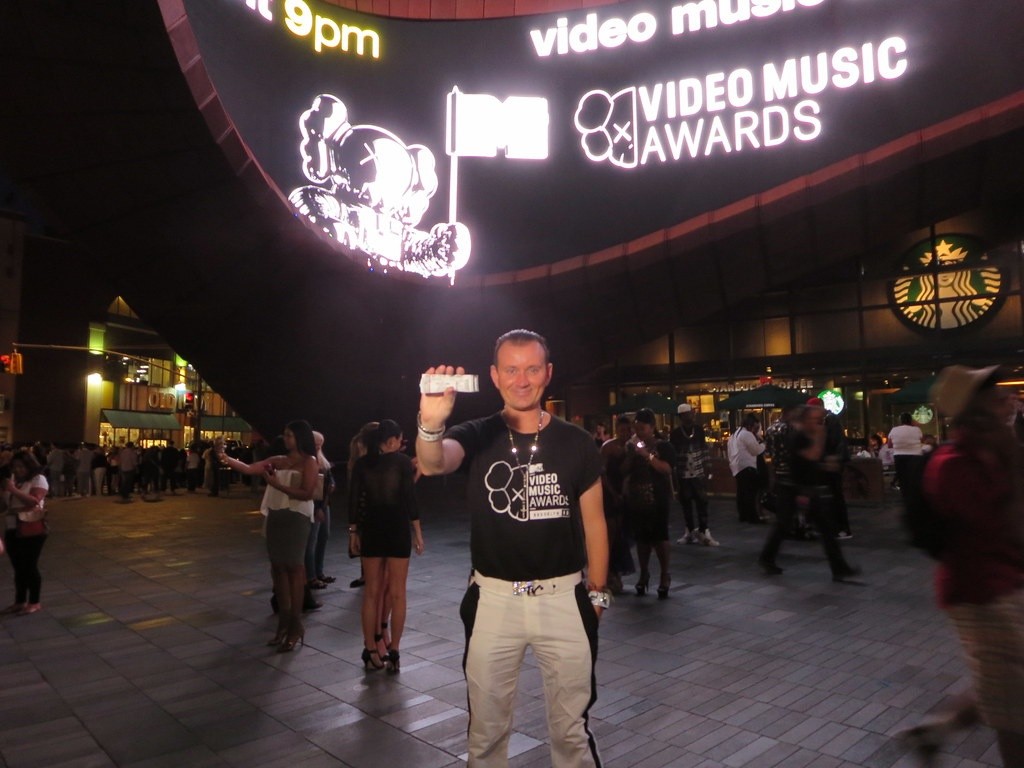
[417,410,446,441]
[219,452,228,465]
[646,451,655,462]
[587,590,611,609]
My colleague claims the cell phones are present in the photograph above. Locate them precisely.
[220,434,226,441]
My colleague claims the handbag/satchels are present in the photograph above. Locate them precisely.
[17,497,48,534]
[289,472,324,500]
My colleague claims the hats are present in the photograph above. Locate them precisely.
[928,363,1002,419]
[678,403,691,415]
[799,398,827,415]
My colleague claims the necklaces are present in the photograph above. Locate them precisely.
[501,409,543,478]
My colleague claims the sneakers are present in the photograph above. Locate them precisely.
[677,527,698,545]
[696,528,720,547]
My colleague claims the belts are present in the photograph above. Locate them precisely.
[474,570,582,597]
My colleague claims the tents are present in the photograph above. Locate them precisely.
[884,375,941,444]
[602,392,683,416]
[714,384,808,435]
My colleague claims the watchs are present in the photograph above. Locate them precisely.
[347,527,357,534]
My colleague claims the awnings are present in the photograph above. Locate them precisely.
[199,415,253,432]
[100,406,182,431]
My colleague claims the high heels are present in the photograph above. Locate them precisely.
[373,632,389,662]
[634,574,649,595]
[388,649,400,672]
[361,647,385,671]
[267,625,290,647]
[381,623,392,651]
[657,575,672,599]
[276,623,305,652]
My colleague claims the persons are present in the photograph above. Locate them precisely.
[214,419,337,653]
[726,412,768,524]
[757,394,865,582]
[591,403,719,598]
[0,450,50,615]
[891,363,1024,768]
[869,412,939,529]
[414,330,619,768]
[0,437,264,497]
[346,420,425,676]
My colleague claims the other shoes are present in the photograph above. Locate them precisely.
[20,602,41,615]
[307,579,328,590]
[5,602,28,615]
[755,555,783,575]
[836,531,854,540]
[301,601,323,613]
[831,564,860,582]
[318,576,336,583]
[349,578,366,588]
[891,720,943,768]
